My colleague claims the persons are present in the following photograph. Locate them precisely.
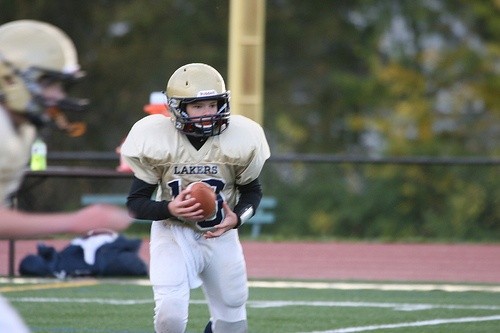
[0,20,136,333]
[119,63,271,333]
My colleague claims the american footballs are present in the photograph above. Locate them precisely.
[181,181,218,220]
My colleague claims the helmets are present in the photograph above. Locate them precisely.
[0,19,90,137]
[167,62,231,136]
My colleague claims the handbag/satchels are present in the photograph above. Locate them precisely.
[56,228,147,278]
